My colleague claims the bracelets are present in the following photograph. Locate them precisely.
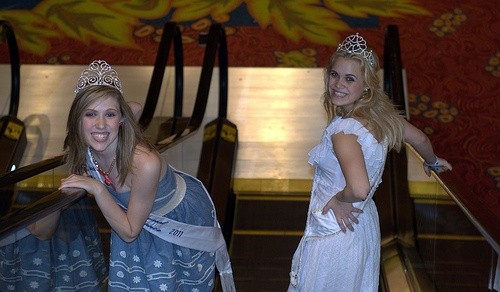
[425,154,440,172]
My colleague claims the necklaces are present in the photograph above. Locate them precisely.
[88,146,120,186]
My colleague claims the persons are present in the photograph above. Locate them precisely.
[59,60,235,292]
[0,195,109,292]
[285,32,452,292]
